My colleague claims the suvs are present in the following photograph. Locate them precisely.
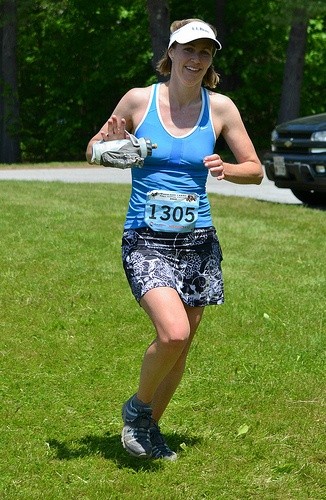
[264,112,326,209]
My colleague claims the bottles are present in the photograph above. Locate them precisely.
[90,129,158,170]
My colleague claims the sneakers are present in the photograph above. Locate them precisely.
[121,393,152,459]
[153,419,177,462]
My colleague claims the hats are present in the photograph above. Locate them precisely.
[168,22,221,58]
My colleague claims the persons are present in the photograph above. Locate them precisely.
[86,19,264,464]
